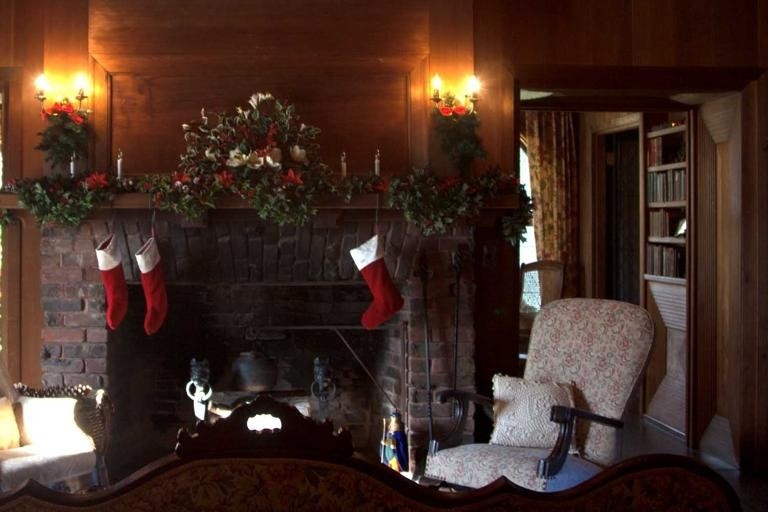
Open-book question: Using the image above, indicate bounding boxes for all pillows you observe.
[490,371,582,456]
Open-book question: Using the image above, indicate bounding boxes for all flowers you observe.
[135,89,336,226]
[42,96,91,155]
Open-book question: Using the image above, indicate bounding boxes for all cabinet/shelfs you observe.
[629,108,715,461]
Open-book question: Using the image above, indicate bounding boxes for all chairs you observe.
[429,297,659,494]
[0,370,121,498]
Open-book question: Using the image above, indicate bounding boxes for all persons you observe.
[380,413,408,473]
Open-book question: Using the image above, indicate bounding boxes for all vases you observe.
[41,148,88,180]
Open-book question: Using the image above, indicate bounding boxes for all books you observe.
[645,137,686,277]
